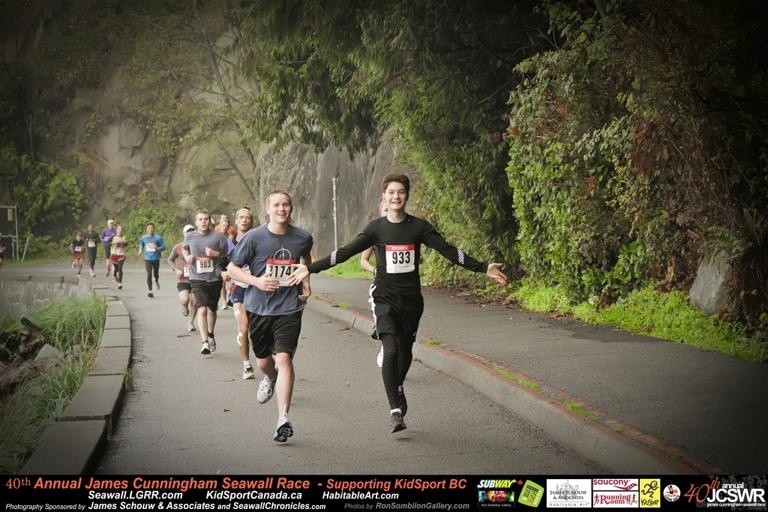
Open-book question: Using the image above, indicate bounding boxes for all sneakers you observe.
[399,387,407,416]
[201,337,216,353]
[243,367,255,380]
[257,369,277,404]
[389,411,406,434]
[273,421,293,443]
[187,323,195,331]
[222,300,228,310]
[148,292,154,297]
[182,308,188,317]
[155,282,160,291]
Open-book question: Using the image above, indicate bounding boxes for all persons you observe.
[68,209,235,355]
[285,171,508,434]
[359,199,389,368]
[215,205,255,381]
[226,188,313,444]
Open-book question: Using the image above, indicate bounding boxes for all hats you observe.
[183,223,195,232]
